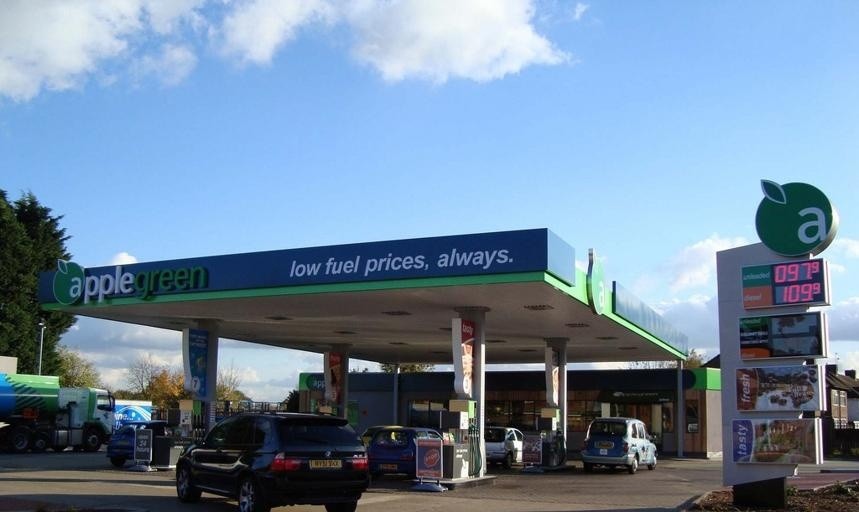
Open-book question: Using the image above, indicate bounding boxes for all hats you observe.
[486,426,523,469]
[106,420,177,467]
[176,410,370,512]
[361,425,444,477]
[580,416,657,474]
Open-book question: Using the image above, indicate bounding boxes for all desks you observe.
[38,318,48,375]
